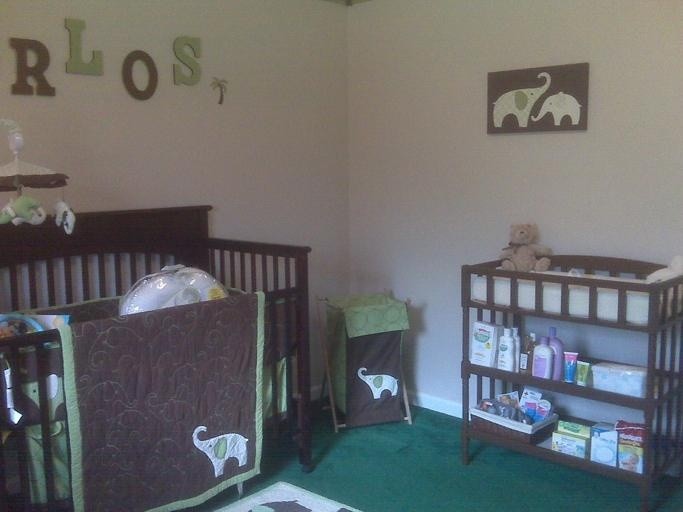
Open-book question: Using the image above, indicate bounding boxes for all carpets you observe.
[212,479,362,512]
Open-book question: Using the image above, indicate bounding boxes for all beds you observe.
[0,206,312,512]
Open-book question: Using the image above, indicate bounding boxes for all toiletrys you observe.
[562,352,579,384]
[576,360,590,386]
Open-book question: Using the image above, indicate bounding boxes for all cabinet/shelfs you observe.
[458,254,682,511]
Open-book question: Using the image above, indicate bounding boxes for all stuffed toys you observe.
[0,310,46,337]
[498,223,552,272]
[0,118,76,235]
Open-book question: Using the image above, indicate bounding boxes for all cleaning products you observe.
[497,327,564,380]
